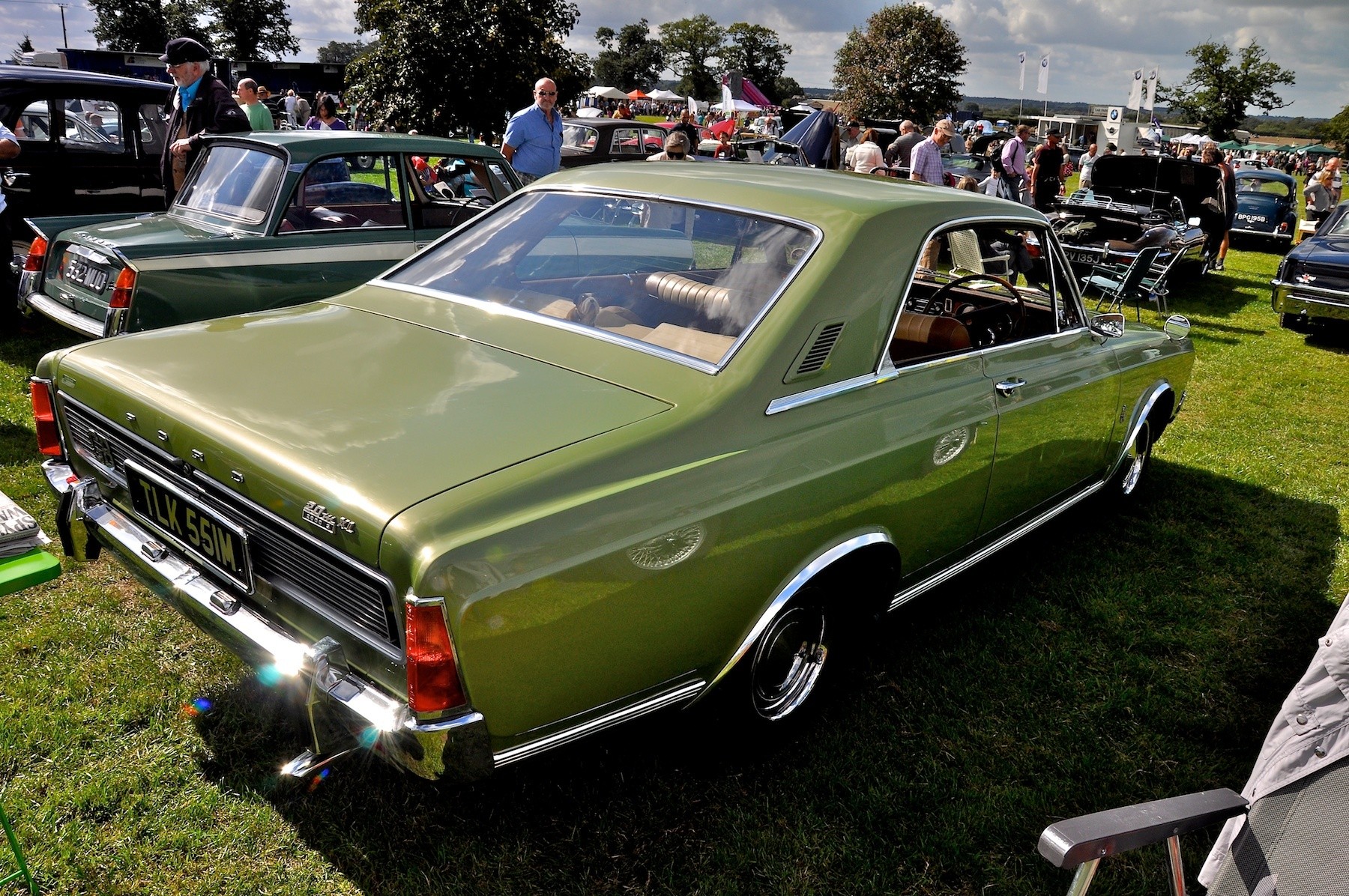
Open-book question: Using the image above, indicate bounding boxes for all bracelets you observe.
[1310,204,1313,205]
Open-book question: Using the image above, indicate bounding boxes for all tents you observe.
[710,99,763,112]
[587,86,686,104]
[1169,132,1339,156]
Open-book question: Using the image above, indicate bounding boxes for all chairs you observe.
[1037,592,1349,896]
[312,205,360,227]
[1096,245,1189,316]
[892,312,971,355]
[948,229,1014,284]
[361,219,383,227]
[643,269,751,332]
[1079,246,1162,322]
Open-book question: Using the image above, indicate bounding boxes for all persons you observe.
[304,96,347,131]
[498,77,563,187]
[1142,144,1343,272]
[280,81,301,126]
[66,99,115,143]
[311,91,327,117]
[296,96,311,126]
[15,118,26,137]
[821,107,1129,293]
[335,91,345,112]
[408,129,507,197]
[257,86,281,130]
[599,99,783,242]
[237,78,274,131]
[0,120,37,336]
[160,38,253,212]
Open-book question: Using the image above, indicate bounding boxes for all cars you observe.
[1025,148,1298,285]
[1271,200,1349,329]
[0,65,1041,341]
[31,159,1195,790]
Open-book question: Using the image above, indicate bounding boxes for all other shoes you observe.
[1215,263,1224,270]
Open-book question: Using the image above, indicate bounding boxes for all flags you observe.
[1126,67,1142,110]
[1144,68,1157,111]
[1037,54,1049,94]
[1019,51,1025,91]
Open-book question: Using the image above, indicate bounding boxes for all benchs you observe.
[212,203,294,233]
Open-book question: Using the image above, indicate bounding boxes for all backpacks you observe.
[990,140,1018,172]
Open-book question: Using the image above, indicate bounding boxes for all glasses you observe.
[539,91,557,96]
[667,150,684,159]
[847,129,854,131]
[164,63,184,68]
[1327,177,1333,180]
[939,130,951,142]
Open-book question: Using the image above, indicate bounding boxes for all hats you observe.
[1047,129,1062,137]
[158,38,210,64]
[257,86,271,97]
[90,113,103,119]
[936,119,955,138]
[847,121,860,128]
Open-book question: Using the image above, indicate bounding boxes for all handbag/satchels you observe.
[1063,156,1073,177]
